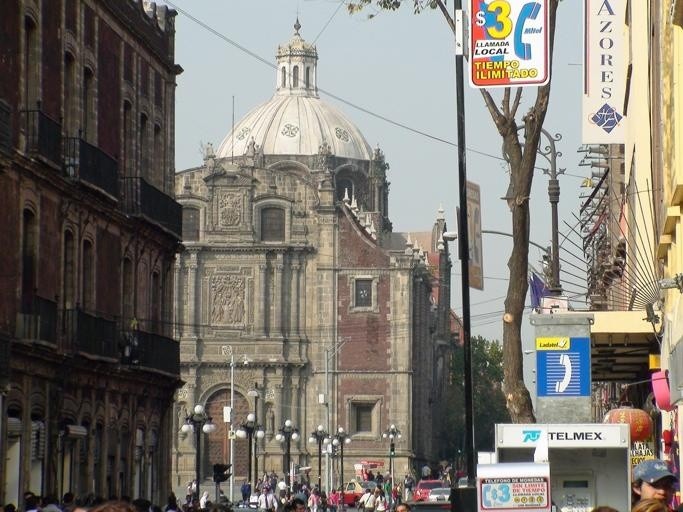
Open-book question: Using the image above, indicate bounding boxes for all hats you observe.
[633,459,680,485]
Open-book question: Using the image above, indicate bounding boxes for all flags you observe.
[529,274,533,310]
[532,272,551,309]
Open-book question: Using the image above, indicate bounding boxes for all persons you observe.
[630,499,673,511]
[630,457,678,505]
[1,460,463,512]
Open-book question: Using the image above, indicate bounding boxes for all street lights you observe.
[331,425,352,507]
[306,424,334,497]
[179,401,216,509]
[381,424,403,504]
[273,417,302,502]
[233,414,268,496]
[229,352,248,504]
[442,229,564,294]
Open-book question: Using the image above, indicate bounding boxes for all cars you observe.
[325,475,387,507]
[412,473,469,507]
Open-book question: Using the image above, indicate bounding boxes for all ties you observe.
[264,495,268,509]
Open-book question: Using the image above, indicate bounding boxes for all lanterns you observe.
[601,400,654,447]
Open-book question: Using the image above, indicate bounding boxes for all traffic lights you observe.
[390,442,396,457]
[211,462,233,482]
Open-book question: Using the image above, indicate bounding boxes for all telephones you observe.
[550,469,597,512]
